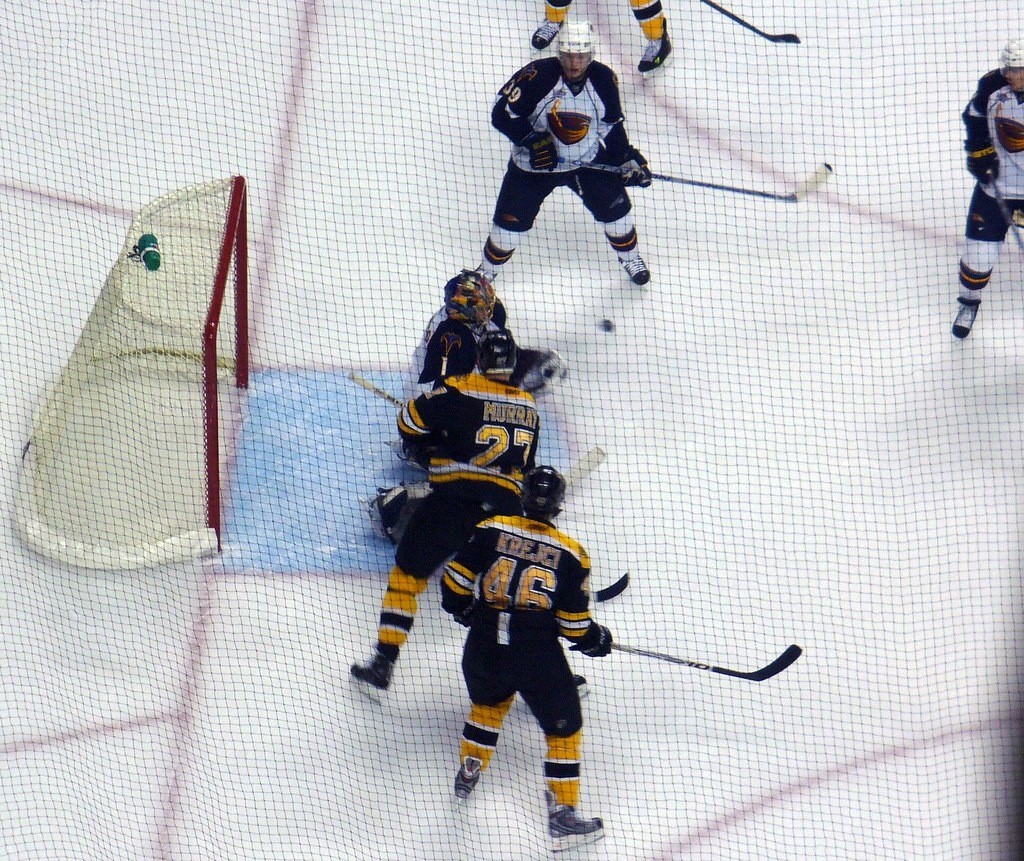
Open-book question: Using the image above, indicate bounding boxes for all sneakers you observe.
[637,34,674,77]
[351,665,391,696]
[384,437,428,471]
[546,792,605,851]
[473,261,498,283]
[454,756,482,806]
[952,304,979,342]
[573,674,591,696]
[532,19,563,53]
[360,487,407,545]
[618,254,650,290]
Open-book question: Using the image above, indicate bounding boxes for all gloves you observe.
[524,131,558,171]
[622,167,652,186]
[964,138,999,185]
[569,620,613,657]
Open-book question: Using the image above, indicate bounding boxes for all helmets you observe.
[523,465,566,517]
[444,268,495,322]
[558,21,594,53]
[476,329,516,375]
[1000,38,1024,67]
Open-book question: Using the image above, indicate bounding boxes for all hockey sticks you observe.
[986,169,1024,251]
[611,643,804,683]
[348,371,607,490]
[699,0,801,43]
[540,153,833,204]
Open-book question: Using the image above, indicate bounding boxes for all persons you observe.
[532,0,672,80]
[950,38,1024,338]
[439,466,613,852]
[409,268,566,404]
[350,330,540,704]
[464,17,653,299]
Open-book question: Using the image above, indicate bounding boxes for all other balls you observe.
[600,318,615,332]
